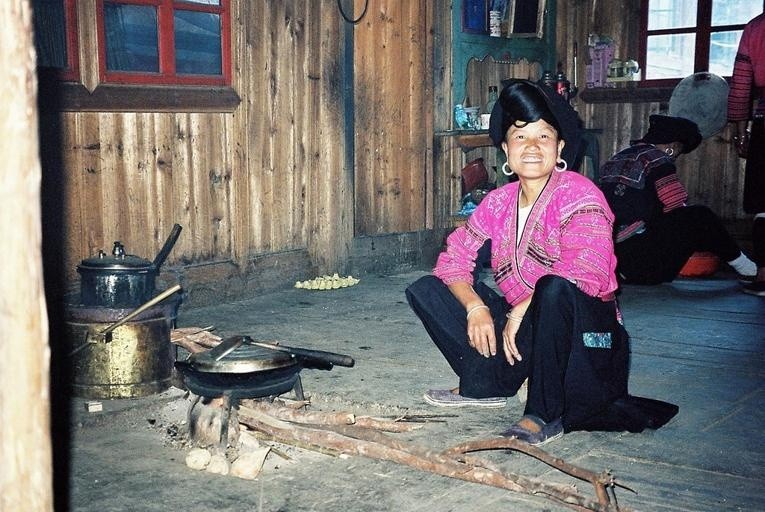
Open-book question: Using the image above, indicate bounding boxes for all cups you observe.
[480,114,492,129]
[606,57,638,76]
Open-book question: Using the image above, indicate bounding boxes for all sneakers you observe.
[739,268,765,297]
[424,387,507,409]
[498,414,564,448]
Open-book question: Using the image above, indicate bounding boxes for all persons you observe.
[405,78,680,449]
[727,11,765,297]
[598,115,759,286]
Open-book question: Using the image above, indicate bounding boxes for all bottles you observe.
[544,73,566,95]
[486,86,499,114]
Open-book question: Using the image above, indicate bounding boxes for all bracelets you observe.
[734,132,749,144]
[506,313,523,322]
[466,305,490,320]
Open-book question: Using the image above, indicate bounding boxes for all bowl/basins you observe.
[680,251,721,277]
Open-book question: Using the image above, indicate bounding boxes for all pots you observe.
[75,242,160,307]
[172,346,331,388]
